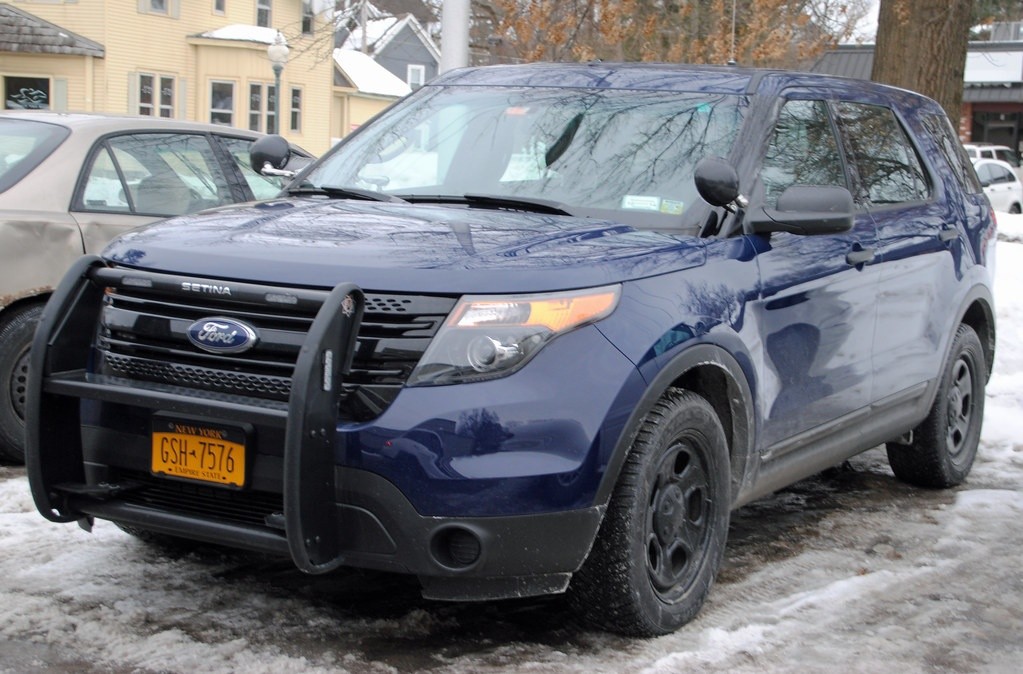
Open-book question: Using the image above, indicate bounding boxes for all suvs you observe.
[21,60,998,638]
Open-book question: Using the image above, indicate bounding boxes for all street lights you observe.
[268,29,293,136]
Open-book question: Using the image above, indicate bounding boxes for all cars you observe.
[0,110,328,467]
[962,158,1022,214]
[963,142,1021,177]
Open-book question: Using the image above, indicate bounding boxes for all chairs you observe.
[137,172,189,215]
